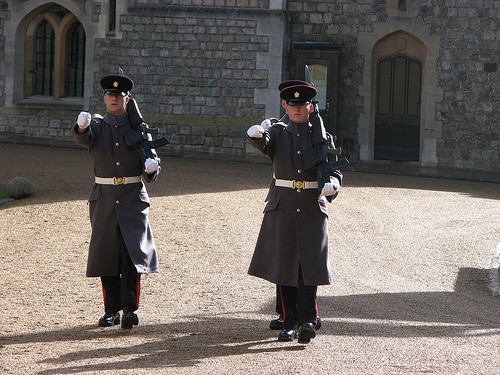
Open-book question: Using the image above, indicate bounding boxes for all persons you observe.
[246,80,343,344]
[72,74,161,328]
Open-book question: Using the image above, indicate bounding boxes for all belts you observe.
[94,176,141,185]
[273,173,277,179]
[275,179,319,189]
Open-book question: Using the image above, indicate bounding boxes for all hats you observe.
[278,80,311,91]
[100,74,134,96]
[280,86,317,105]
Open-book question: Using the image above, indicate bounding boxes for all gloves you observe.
[247,125,264,138]
[261,119,272,131]
[145,158,158,174]
[77,111,91,129]
[321,182,338,197]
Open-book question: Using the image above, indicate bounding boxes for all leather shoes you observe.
[278,329,298,341]
[270,317,284,329]
[121,313,138,329]
[99,312,120,326]
[316,318,321,330]
[298,322,315,343]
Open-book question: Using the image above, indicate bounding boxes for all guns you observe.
[119,65,170,165]
[305,64,351,188]
